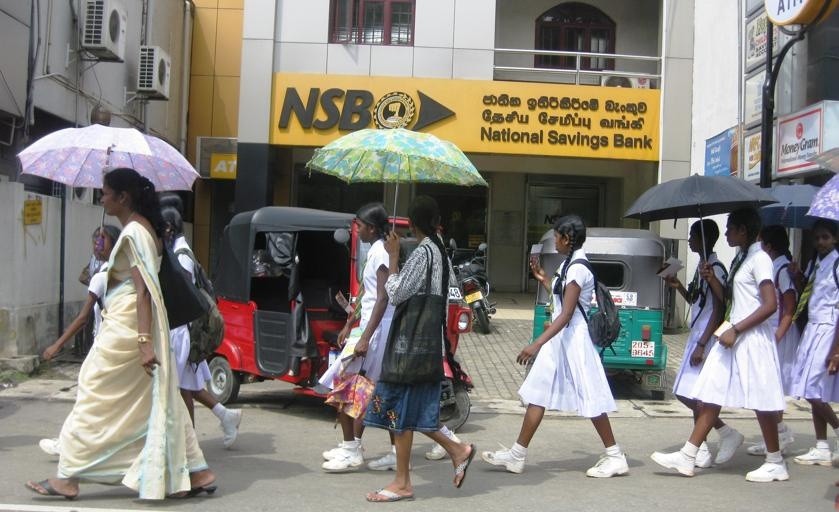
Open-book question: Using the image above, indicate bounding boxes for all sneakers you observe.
[220,410,243,447]
[369,452,411,472]
[426,434,459,460]
[746,428,793,482]
[323,443,364,470]
[483,450,524,474]
[716,428,744,464]
[39,439,62,456]
[651,451,695,477]
[794,437,839,466]
[696,448,711,467]
[586,455,628,478]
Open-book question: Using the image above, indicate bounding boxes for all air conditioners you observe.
[83,0,127,64]
[136,46,172,103]
[600,70,650,89]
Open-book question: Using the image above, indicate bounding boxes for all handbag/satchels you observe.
[563,259,621,346]
[777,263,809,329]
[175,247,224,361]
[157,241,207,329]
[379,293,447,385]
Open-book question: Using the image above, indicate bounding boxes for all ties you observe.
[355,256,369,318]
[725,255,747,321]
[792,265,818,321]
[548,268,557,313]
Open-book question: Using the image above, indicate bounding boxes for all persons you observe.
[20,169,240,500]
[650,206,839,482]
[320,197,476,500]
[481,215,629,478]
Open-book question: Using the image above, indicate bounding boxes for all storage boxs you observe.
[455,233,485,248]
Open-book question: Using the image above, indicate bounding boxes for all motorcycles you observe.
[453,241,497,336]
[528,225,668,403]
[210,208,475,431]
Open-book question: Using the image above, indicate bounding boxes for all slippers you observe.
[24,481,78,501]
[366,488,414,502]
[172,488,217,500]
[453,444,477,488]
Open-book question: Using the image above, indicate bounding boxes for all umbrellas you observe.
[805,173,839,222]
[304,125,490,234]
[755,182,823,267]
[15,123,203,255]
[622,174,781,263]
